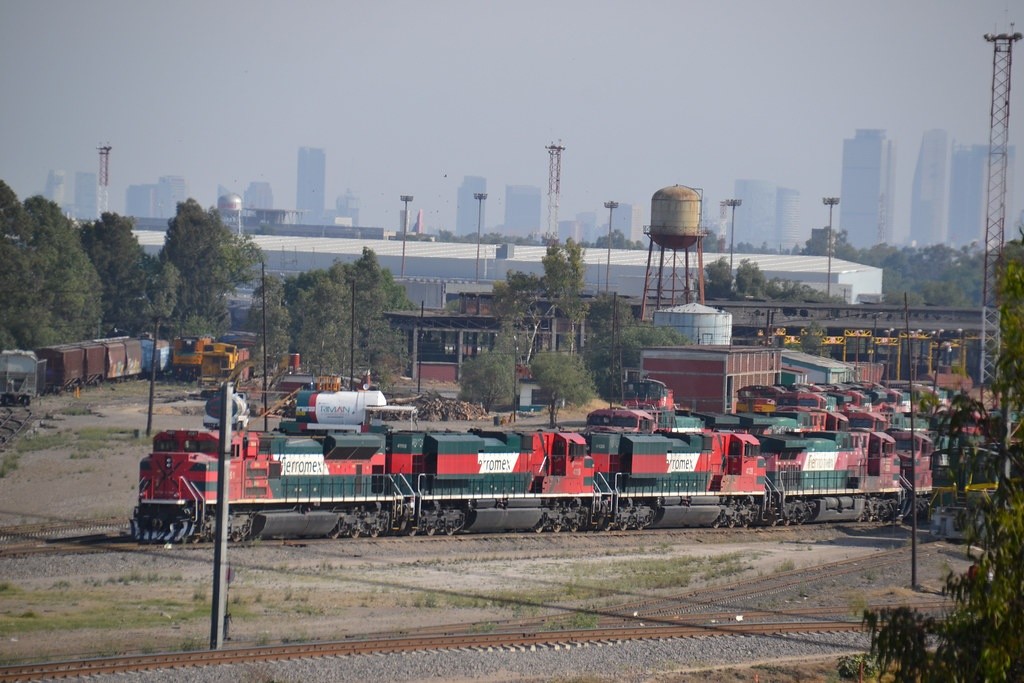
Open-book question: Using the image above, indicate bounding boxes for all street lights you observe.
[474,192,488,283]
[604,200,619,295]
[724,199,743,294]
[824,196,841,299]
[399,194,414,278]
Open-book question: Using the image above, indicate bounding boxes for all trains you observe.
[127,378,1024,545]
[0,331,172,409]
